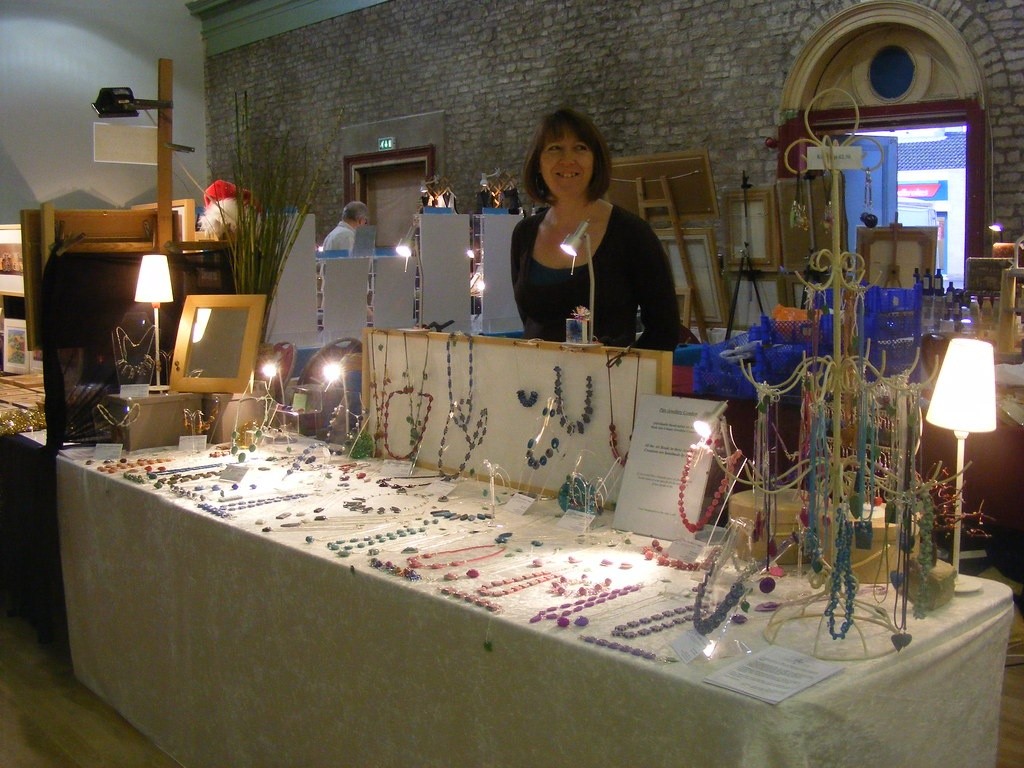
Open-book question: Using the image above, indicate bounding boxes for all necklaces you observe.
[690,286,920,651]
[87,325,741,661]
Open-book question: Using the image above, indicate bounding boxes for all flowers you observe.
[572,305,592,323]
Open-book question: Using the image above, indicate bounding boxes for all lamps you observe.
[324,351,353,434]
[261,351,286,428]
[479,172,488,187]
[91,86,174,119]
[988,223,1005,242]
[419,178,429,194]
[396,225,418,257]
[133,254,175,391]
[558,219,596,343]
[924,336,997,593]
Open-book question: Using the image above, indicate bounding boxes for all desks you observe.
[670,366,1024,583]
[56,444,1013,768]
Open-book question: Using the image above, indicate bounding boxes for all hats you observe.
[197,179,266,240]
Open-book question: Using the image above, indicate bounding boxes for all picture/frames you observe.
[131,199,195,253]
[3,318,30,375]
[786,273,851,309]
[857,225,937,288]
[655,227,730,329]
[168,294,268,392]
[0,224,24,297]
[722,272,786,331]
[606,147,720,222]
[724,184,780,272]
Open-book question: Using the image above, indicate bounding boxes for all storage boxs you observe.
[692,282,921,400]
[819,510,920,583]
[205,393,269,445]
[108,391,204,451]
[0,372,45,415]
[728,488,809,563]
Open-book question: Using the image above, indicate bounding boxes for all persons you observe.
[322,201,369,251]
[203,179,261,242]
[511,109,679,351]
